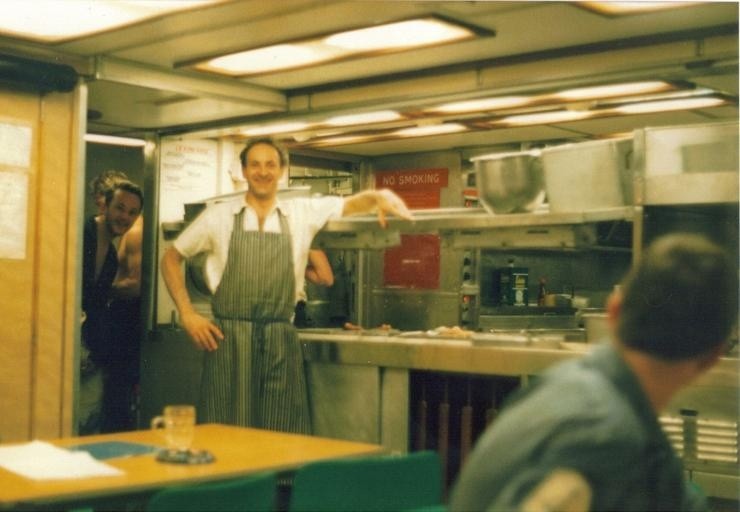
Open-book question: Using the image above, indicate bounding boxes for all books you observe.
[541,136,633,212]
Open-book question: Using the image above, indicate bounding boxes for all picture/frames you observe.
[0,423,386,512]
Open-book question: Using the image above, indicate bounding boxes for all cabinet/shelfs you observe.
[538,276,547,307]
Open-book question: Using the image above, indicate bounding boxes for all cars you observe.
[151,405,197,453]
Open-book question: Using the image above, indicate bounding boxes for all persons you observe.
[293,248,337,327]
[445,232,737,511]
[157,136,416,431]
[77,179,146,437]
[88,169,147,436]
